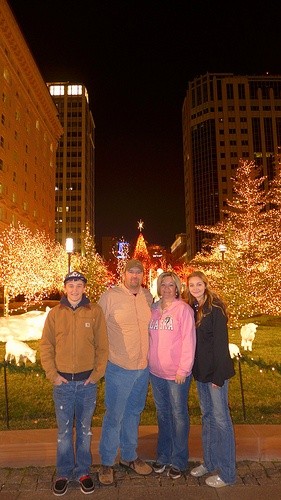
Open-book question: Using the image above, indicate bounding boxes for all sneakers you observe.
[119,457,153,475]
[98,465,114,485]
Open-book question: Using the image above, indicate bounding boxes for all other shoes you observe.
[79,475,95,494]
[169,468,182,479]
[191,465,208,477]
[153,463,166,473]
[53,477,69,496]
[205,475,229,488]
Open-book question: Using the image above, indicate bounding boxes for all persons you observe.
[147,271,196,479]
[181,272,237,488]
[97,259,154,485]
[39,271,109,496]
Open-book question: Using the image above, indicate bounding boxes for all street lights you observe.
[219,243,227,262]
[66,237,74,275]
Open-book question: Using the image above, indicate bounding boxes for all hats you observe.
[126,259,143,271]
[63,271,85,282]
